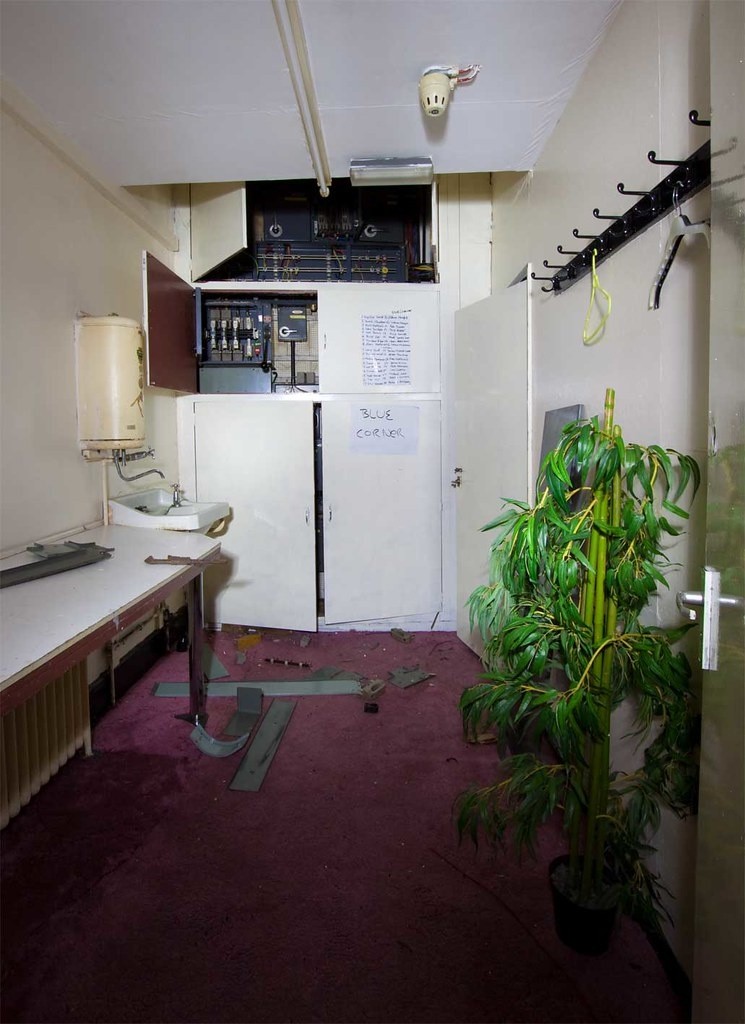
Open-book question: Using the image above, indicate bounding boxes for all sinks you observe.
[108,487,230,536]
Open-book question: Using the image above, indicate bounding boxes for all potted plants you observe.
[450,387,703,950]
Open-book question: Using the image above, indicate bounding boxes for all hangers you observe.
[581,249,612,344]
[648,180,712,307]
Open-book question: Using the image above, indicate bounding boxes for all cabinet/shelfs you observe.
[140,179,539,673]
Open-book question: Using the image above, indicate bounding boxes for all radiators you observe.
[0,657,95,831]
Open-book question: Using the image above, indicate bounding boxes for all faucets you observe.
[111,444,166,482]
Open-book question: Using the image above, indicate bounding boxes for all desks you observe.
[0,524,223,731]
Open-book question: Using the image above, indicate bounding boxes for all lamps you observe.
[349,156,433,187]
